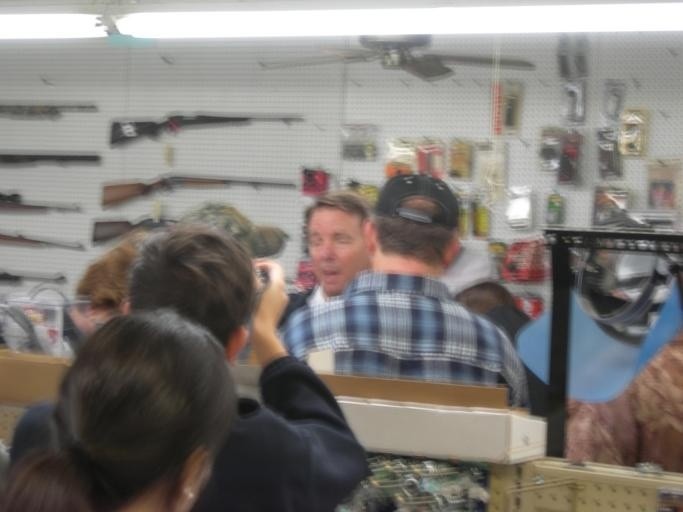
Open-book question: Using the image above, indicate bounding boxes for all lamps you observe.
[111,2,681,41]
[3,12,111,39]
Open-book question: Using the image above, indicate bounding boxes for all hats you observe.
[512,258,683,404]
[373,172,459,228]
[179,205,285,257]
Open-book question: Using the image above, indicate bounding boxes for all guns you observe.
[0,104,98,119]
[100,175,295,209]
[90,216,288,247]
[0,232,82,252]
[0,272,66,285]
[0,150,100,165]
[110,113,306,143]
[0,194,80,218]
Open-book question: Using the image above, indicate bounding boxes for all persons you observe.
[563,89,583,120]
[133,222,375,512]
[450,278,566,417]
[2,311,243,511]
[1,233,156,369]
[167,203,290,312]
[275,192,379,333]
[606,91,622,120]
[274,171,534,413]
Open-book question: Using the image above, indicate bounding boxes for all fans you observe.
[256,35,535,85]
